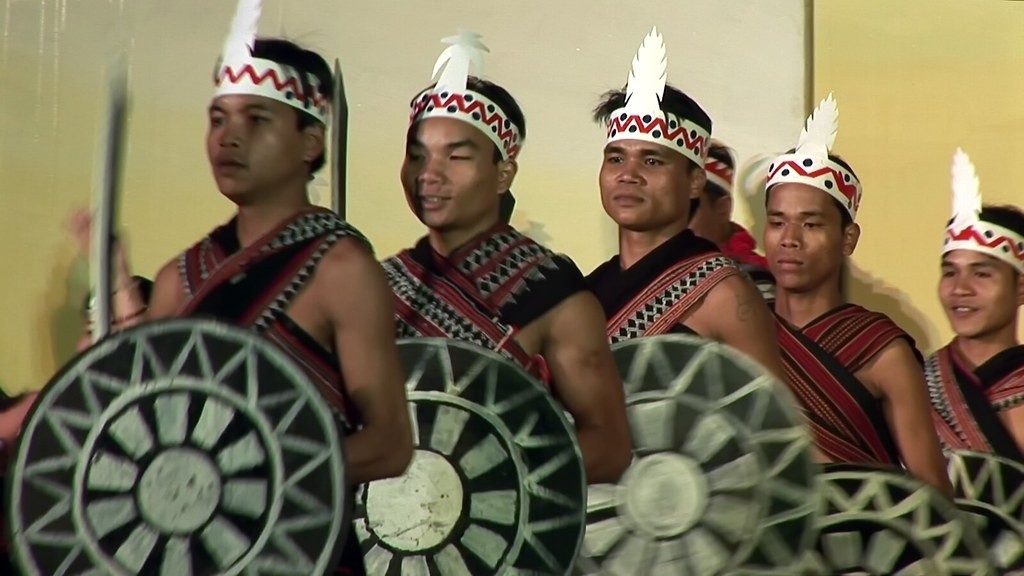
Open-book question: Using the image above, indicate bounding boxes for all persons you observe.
[764,93,954,500]
[688,138,778,313]
[584,25,784,386]
[379,27,632,482]
[0,0,413,576]
[922,145,1024,467]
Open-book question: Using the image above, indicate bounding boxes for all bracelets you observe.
[111,306,149,325]
[110,280,141,296]
